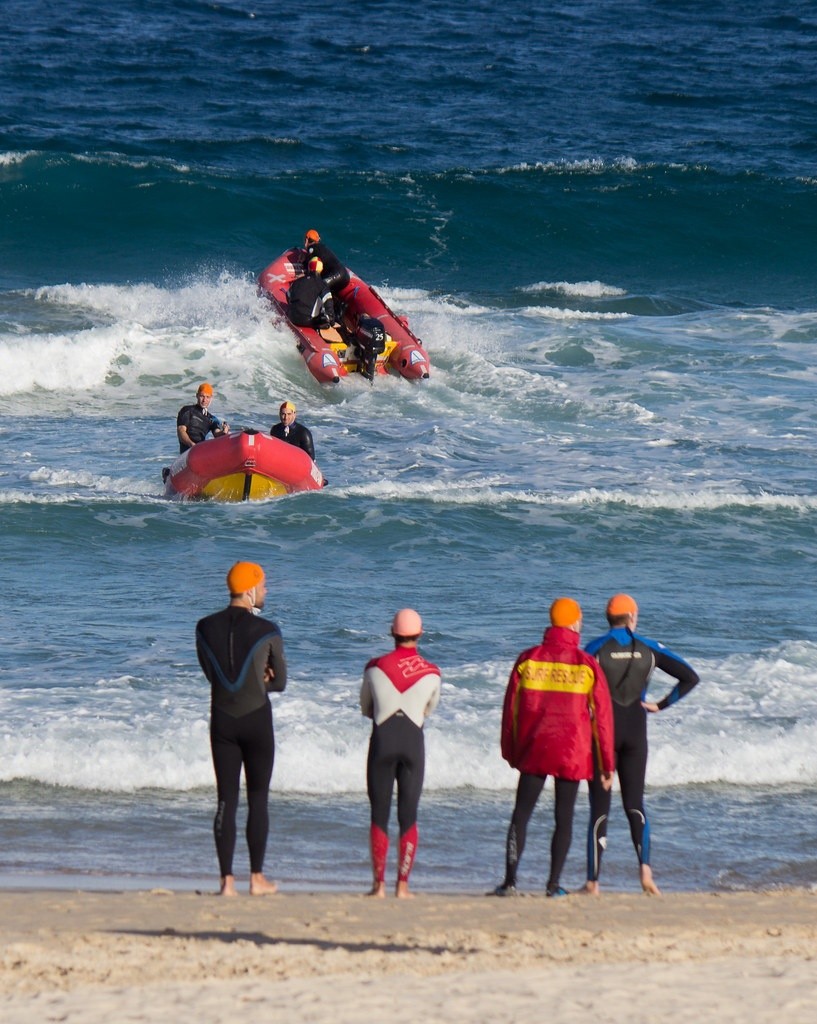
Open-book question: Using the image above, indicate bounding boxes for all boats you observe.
[161,427,330,504]
[257,246,431,385]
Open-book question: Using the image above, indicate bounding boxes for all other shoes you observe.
[546,886,570,896]
[494,885,516,896]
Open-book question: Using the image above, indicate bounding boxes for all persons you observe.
[360,610,441,899]
[574,594,700,898]
[270,402,315,462]
[196,561,287,896]
[287,230,350,329]
[177,384,230,455]
[485,597,614,897]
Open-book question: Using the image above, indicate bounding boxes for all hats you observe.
[307,256,324,274]
[549,597,582,628]
[391,608,422,638]
[361,313,370,318]
[198,383,213,396]
[305,230,320,242]
[280,401,296,412]
[226,561,264,594]
[606,594,638,615]
